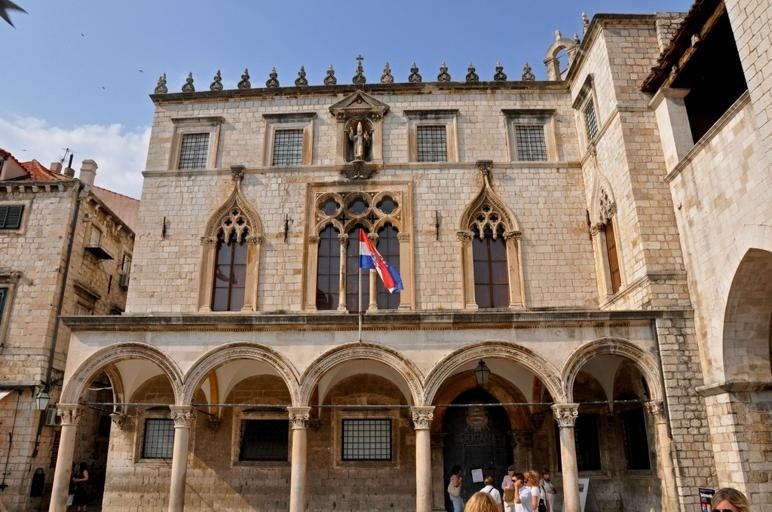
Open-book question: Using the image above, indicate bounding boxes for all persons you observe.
[71,462,89,512]
[711,489,752,512]
[448,465,558,512]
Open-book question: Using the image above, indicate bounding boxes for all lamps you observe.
[33,379,64,409]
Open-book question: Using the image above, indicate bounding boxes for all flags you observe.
[359,227,404,294]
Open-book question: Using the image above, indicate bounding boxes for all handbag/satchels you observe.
[538,499,546,512]
[447,482,461,496]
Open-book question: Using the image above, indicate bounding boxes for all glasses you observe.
[512,480,515,483]
[524,479,528,483]
[711,509,733,512]
